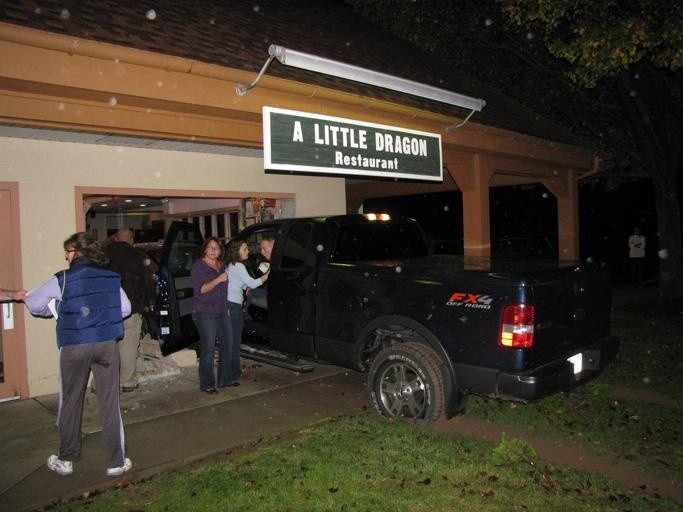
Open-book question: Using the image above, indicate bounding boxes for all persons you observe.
[260,238,274,261]
[90,228,152,395]
[25,231,134,477]
[225,239,268,382]
[627,225,649,291]
[190,237,239,393]
[0,287,28,303]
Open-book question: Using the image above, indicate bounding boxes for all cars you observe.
[130,240,207,339]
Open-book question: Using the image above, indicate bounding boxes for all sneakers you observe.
[200,388,218,395]
[44,453,75,477]
[106,456,133,478]
[121,383,139,393]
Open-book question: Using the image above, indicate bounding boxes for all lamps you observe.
[233,44,488,134]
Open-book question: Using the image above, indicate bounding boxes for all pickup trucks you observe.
[146,211,619,422]
